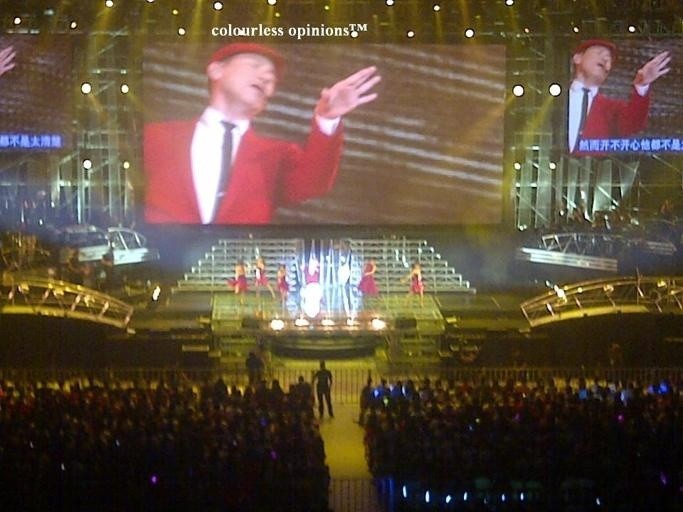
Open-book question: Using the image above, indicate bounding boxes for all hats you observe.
[573,39,619,52]
[207,42,284,83]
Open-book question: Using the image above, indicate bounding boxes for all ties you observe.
[573,88,590,153]
[211,121,236,221]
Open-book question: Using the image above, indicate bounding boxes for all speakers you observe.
[242,318,259,328]
[395,318,416,328]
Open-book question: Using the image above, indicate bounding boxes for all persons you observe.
[399,262,425,308]
[146,42,383,224]
[361,367,683,512]
[522,193,683,273]
[299,255,326,306]
[311,359,337,420]
[277,262,288,303]
[0,229,116,297]
[225,255,249,300]
[253,254,277,301]
[0,45,17,77]
[245,352,264,388]
[0,367,328,512]
[565,39,672,157]
[355,255,384,304]
